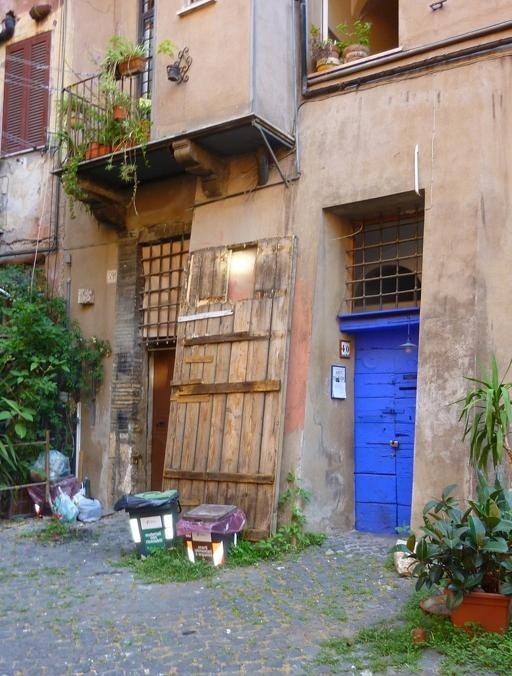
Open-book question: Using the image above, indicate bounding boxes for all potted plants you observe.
[387,355,512,635]
[47,34,182,220]
[310,15,373,74]
